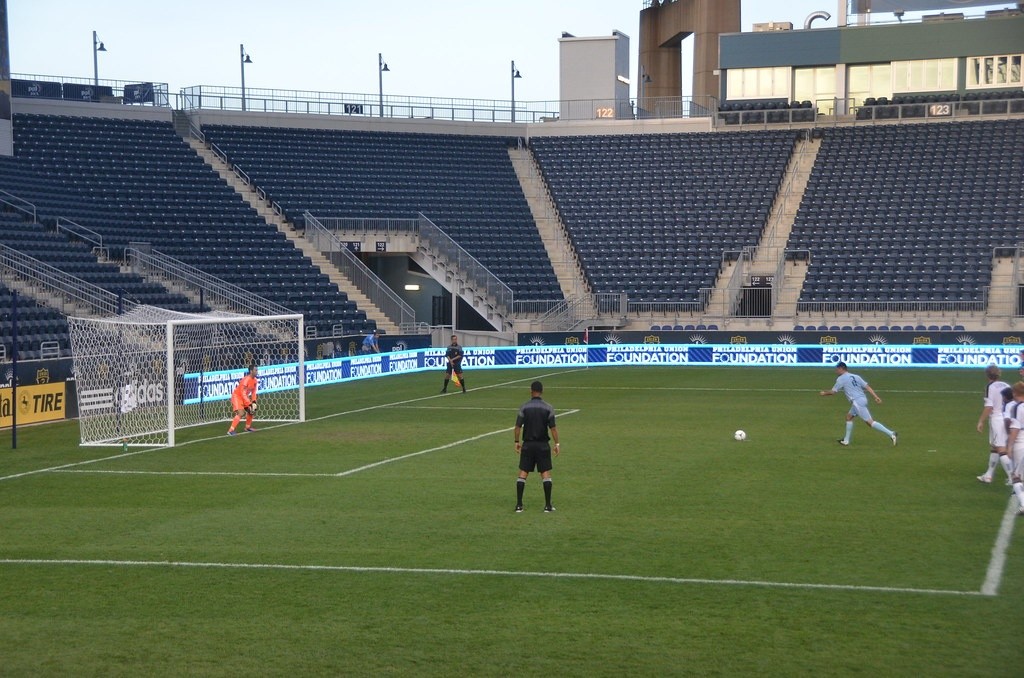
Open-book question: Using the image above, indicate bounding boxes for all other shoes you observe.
[463,389,466,393]
[244,427,257,433]
[440,389,446,393]
[226,431,237,437]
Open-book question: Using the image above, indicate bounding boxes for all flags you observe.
[451,368,461,388]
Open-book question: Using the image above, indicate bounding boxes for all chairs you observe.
[0,92,1024,363]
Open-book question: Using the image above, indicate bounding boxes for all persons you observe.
[820,362,898,446]
[514,380,560,513]
[977,365,1024,514]
[362,330,381,355]
[441,335,467,394]
[227,364,259,436]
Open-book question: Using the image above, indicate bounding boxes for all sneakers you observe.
[544,507,556,512]
[1014,507,1024,515]
[977,475,991,483]
[891,432,898,446]
[515,507,523,512]
[837,439,850,446]
[1004,478,1013,485]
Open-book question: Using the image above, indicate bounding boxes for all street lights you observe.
[240,53,254,113]
[379,63,392,116]
[512,71,522,124]
[640,74,654,111]
[94,40,107,101]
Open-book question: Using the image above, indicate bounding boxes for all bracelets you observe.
[515,441,519,443]
[555,443,559,446]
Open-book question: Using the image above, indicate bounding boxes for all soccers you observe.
[734,430,746,441]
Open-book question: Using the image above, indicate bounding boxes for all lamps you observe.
[405,284,420,291]
